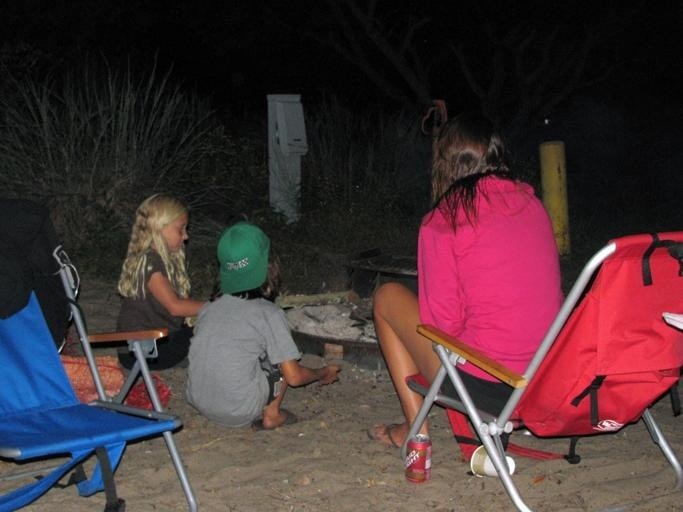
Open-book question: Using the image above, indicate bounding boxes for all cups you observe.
[468,445,515,476]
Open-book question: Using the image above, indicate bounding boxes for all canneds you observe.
[405,434,432,483]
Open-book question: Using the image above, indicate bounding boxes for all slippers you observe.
[252,408,298,432]
[367,424,399,448]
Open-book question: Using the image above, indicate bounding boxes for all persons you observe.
[366,110,566,460]
[185,222,340,432]
[112,192,209,372]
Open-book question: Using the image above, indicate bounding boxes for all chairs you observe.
[399,228,682,512]
[0,195,207,510]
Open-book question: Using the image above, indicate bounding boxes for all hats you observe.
[217,223,271,295]
